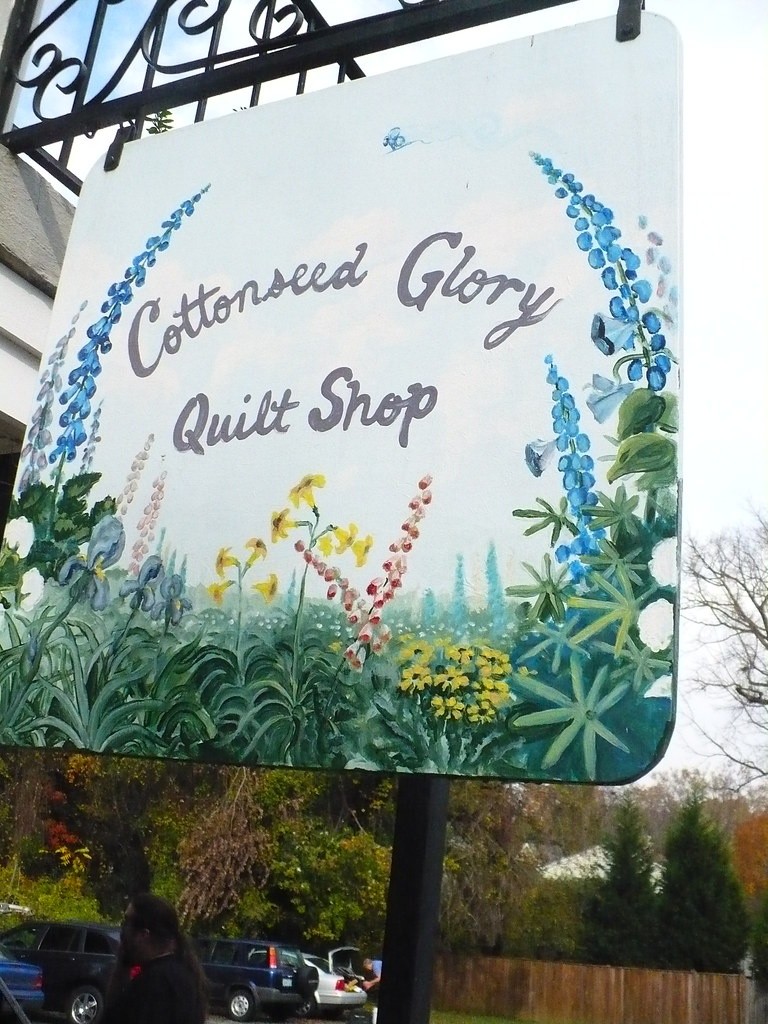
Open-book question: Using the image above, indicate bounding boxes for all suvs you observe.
[0,920,143,1024]
[189,937,320,1024]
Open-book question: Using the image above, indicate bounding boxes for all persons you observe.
[104,893,210,1024]
[363,958,383,991]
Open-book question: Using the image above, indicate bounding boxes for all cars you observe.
[246,945,367,1017]
